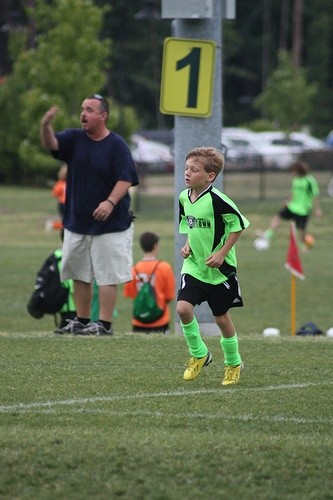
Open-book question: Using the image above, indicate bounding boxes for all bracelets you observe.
[107,199,114,207]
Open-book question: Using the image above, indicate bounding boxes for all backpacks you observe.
[131,261,166,323]
[28,253,70,319]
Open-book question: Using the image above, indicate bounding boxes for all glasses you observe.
[93,94,106,105]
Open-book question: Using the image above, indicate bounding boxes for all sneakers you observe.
[182,352,212,380]
[53,317,89,334]
[74,321,113,335]
[221,363,244,386]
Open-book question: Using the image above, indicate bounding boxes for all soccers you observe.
[251,236,270,252]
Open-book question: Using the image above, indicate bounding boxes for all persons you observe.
[53,229,77,329]
[42,96,139,336]
[255,159,319,250]
[90,280,118,323]
[124,232,176,334]
[176,148,250,384]
[52,166,66,221]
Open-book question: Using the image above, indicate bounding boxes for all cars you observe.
[222,127,333,168]
[130,129,175,162]
